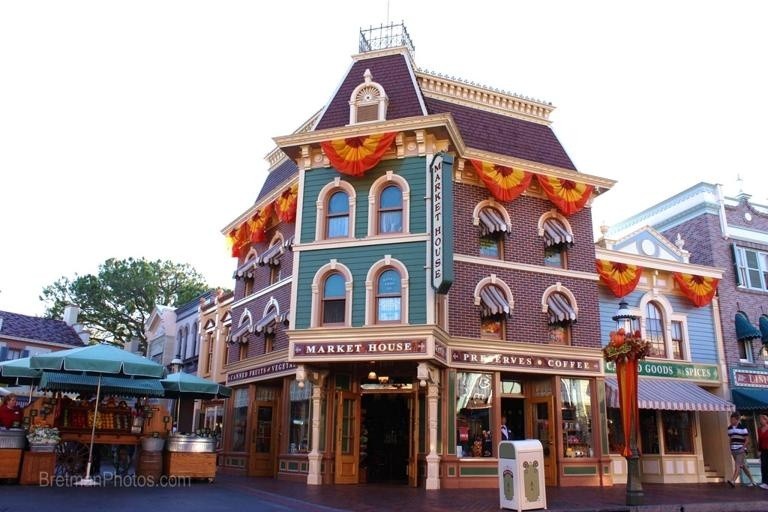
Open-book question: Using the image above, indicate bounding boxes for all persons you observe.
[194,424,221,449]
[728,411,757,487]
[500,415,513,440]
[0,394,23,428]
[472,429,492,457]
[172,421,177,432]
[756,414,768,492]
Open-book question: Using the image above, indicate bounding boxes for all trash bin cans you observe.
[497,439,547,512]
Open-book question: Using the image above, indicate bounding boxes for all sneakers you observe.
[758,483,767,489]
[746,483,756,487]
[727,480,735,487]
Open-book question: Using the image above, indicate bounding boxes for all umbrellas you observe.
[160,367,233,433]
[0,353,42,403]
[35,340,169,478]
